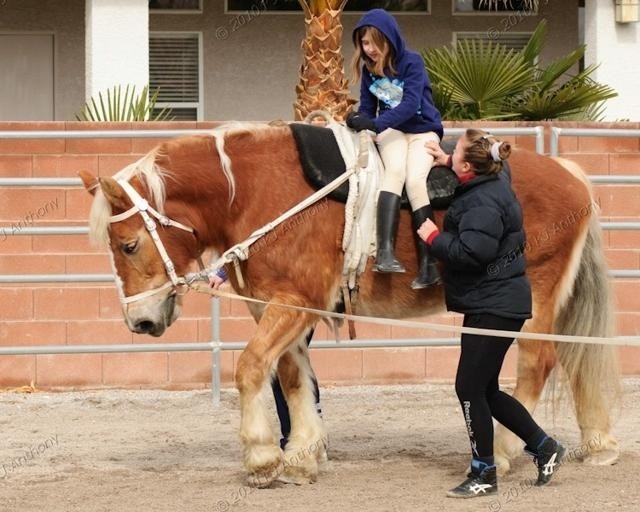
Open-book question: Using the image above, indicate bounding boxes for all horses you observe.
[75,119,627,489]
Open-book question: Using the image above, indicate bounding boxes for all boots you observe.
[410,204,445,290]
[444,455,501,499]
[371,190,407,274]
[522,427,567,487]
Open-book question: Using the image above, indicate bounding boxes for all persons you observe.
[412,126,568,500]
[344,8,447,287]
